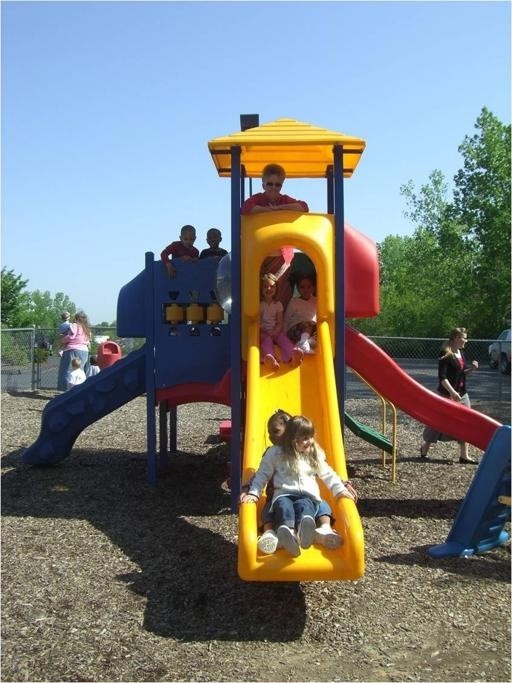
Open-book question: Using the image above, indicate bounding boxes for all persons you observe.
[60,308,103,394]
[160,222,199,317]
[285,275,321,352]
[247,415,357,556]
[241,164,310,308]
[243,407,360,542]
[260,272,302,371]
[198,228,226,325]
[418,325,479,465]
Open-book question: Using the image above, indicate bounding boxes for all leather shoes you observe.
[458,456,479,465]
[420,445,430,460]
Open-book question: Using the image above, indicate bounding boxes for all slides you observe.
[345,323,505,450]
[239,209,367,581]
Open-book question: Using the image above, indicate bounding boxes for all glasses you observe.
[264,181,283,187]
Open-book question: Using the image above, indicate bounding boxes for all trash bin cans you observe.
[97,341,121,369]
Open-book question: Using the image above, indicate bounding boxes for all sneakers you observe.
[265,353,280,371]
[299,515,316,550]
[289,346,304,369]
[295,335,317,355]
[314,526,345,549]
[257,532,278,555]
[276,524,301,558]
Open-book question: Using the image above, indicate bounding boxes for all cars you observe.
[26,334,55,356]
[488,328,511,373]
[92,334,139,351]
